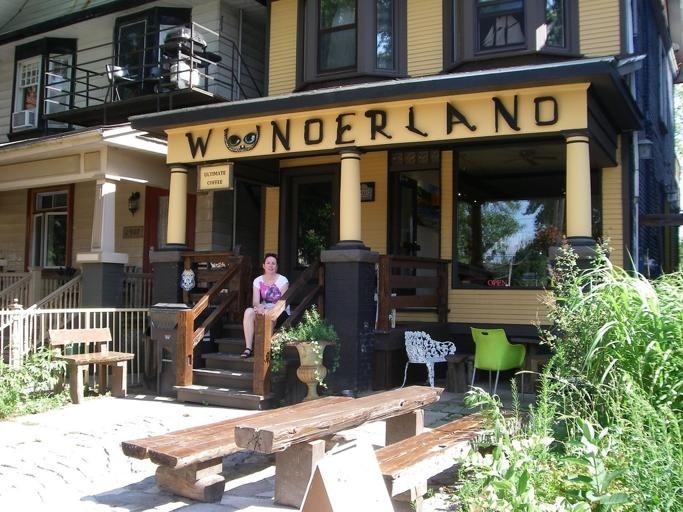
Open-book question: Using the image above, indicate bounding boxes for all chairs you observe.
[101,63,140,103]
[397,323,528,403]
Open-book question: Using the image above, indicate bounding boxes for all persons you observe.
[237,253,290,359]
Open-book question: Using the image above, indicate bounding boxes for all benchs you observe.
[47,325,138,405]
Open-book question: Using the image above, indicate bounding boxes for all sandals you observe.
[240,348,253,358]
